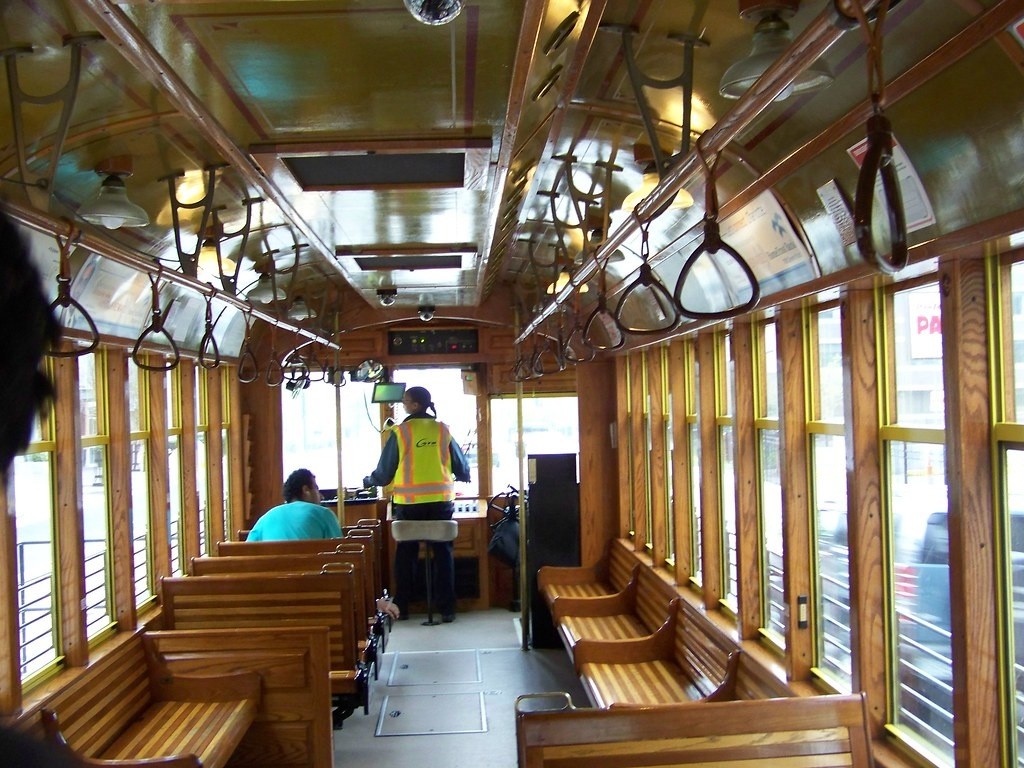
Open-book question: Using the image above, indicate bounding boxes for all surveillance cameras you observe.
[376,287,399,308]
[403,0,465,26]
[418,292,437,321]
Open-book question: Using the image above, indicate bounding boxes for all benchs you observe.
[3,518,396,768]
[515,541,874,768]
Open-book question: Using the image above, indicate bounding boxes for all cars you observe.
[461,443,500,467]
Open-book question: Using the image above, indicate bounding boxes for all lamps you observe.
[546,263,589,294]
[622,162,694,211]
[286,295,317,320]
[576,230,626,264]
[718,8,836,99]
[74,173,150,230]
[196,239,236,274]
[245,273,287,303]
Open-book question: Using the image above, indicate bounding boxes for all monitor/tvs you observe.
[372,382,406,403]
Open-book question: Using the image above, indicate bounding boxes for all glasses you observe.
[402,399,412,403]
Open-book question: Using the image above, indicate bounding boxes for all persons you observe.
[362,385,472,624]
[243,468,400,620]
